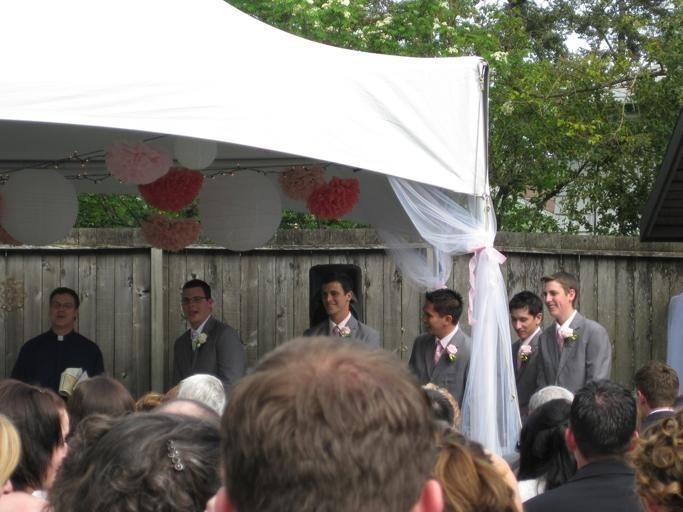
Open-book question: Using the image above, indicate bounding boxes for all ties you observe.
[332,326,341,336]
[191,331,198,351]
[556,328,565,353]
[516,348,523,369]
[433,341,442,366]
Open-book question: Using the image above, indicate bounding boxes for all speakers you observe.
[309,264,362,327]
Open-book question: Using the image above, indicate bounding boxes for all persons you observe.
[511,273,611,428]
[408,288,472,440]
[303,273,380,352]
[174,279,249,404]
[1,335,682,511]
[11,287,104,405]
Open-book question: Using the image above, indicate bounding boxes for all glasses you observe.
[182,296,206,306]
